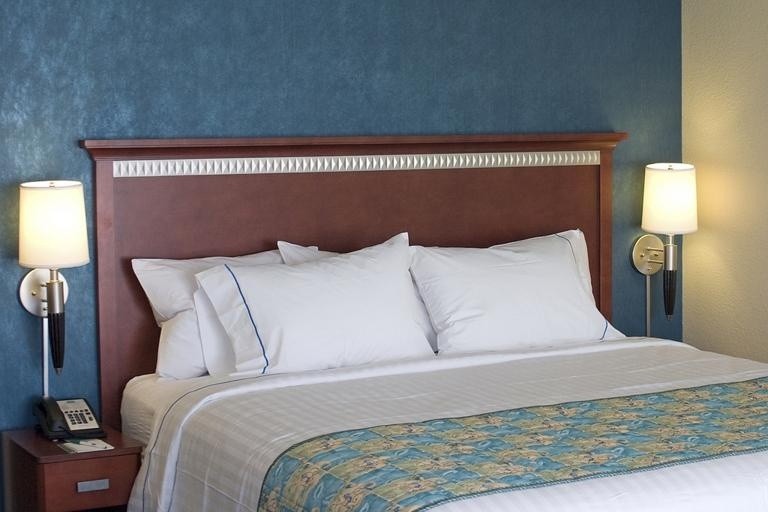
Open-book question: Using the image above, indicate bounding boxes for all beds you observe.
[79,131,766,512]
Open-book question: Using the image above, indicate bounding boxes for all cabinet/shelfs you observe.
[0,426,148,512]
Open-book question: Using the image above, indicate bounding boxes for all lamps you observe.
[630,161,699,338]
[14,177,91,401]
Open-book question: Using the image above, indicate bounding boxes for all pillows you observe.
[408,228,624,361]
[131,244,318,376]
[201,244,436,377]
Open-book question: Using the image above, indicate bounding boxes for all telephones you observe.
[33,396,107,439]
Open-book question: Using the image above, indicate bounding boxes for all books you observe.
[55,438,115,454]
